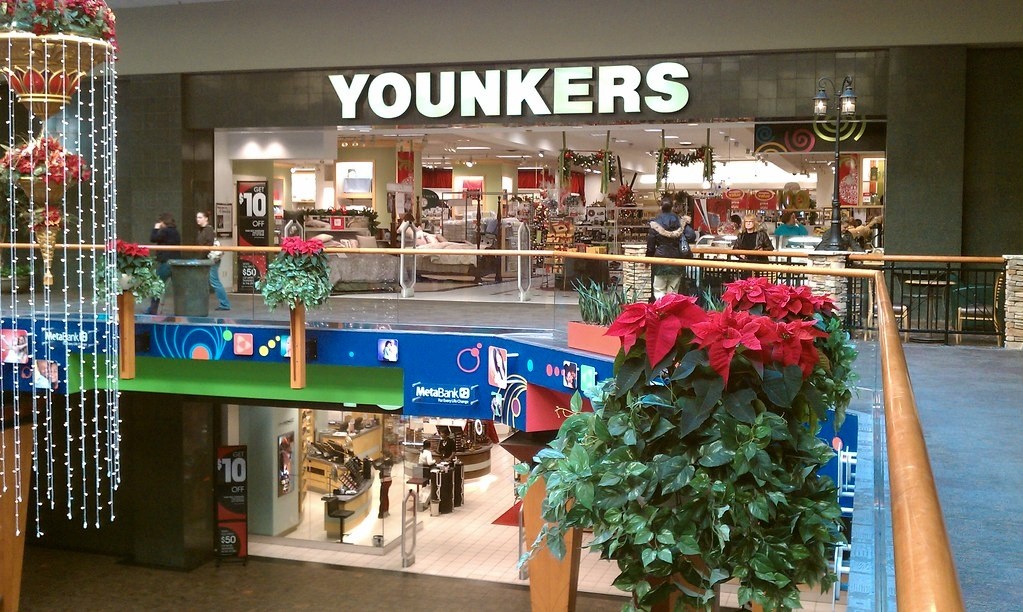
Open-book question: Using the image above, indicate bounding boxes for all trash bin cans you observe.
[168,259,211,317]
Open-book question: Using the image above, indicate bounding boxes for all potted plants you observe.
[0,183,39,295]
[564,275,647,358]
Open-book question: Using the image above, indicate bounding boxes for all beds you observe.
[440,214,518,243]
[302,214,402,293]
[389,194,504,286]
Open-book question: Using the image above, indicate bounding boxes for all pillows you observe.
[416,230,423,239]
[416,239,425,246]
[313,233,333,243]
[427,235,438,243]
[355,234,378,248]
[339,238,350,248]
[323,239,346,247]
[436,233,448,242]
[349,239,359,248]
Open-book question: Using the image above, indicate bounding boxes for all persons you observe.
[733,214,775,279]
[644,203,696,301]
[195,212,231,311]
[340,416,352,432]
[143,213,180,314]
[822,214,862,252]
[369,449,394,518]
[354,417,364,430]
[419,441,439,502]
[773,212,809,248]
[439,428,455,462]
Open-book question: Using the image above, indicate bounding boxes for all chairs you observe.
[954,271,1004,343]
[866,270,911,344]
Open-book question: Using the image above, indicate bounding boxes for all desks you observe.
[902,279,961,343]
[407,477,429,512]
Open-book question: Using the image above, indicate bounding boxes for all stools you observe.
[326,496,356,545]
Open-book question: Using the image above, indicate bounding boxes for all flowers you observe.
[255,234,331,310]
[19,202,78,231]
[91,240,168,302]
[0,125,96,187]
[0,0,122,61]
[488,268,857,612]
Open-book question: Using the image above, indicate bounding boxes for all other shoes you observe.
[214,306,231,311]
[432,499,440,503]
[144,310,157,315]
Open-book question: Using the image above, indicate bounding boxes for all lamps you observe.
[360,136,367,146]
[538,151,544,158]
[370,135,377,146]
[341,138,349,147]
[443,143,478,168]
[351,137,360,146]
[648,136,811,179]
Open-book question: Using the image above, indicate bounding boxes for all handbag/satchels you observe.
[207,236,224,261]
[676,215,693,259]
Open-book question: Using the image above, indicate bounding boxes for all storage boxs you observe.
[639,233,647,242]
[586,247,608,254]
[632,232,640,242]
[343,177,371,193]
[575,243,587,253]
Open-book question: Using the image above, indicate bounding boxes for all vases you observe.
[0,29,118,74]
[114,269,144,292]
[16,173,80,205]
[33,226,61,284]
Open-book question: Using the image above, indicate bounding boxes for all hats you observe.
[438,427,450,436]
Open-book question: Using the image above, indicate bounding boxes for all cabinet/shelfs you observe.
[570,215,616,252]
[616,206,685,257]
[539,242,567,286]
